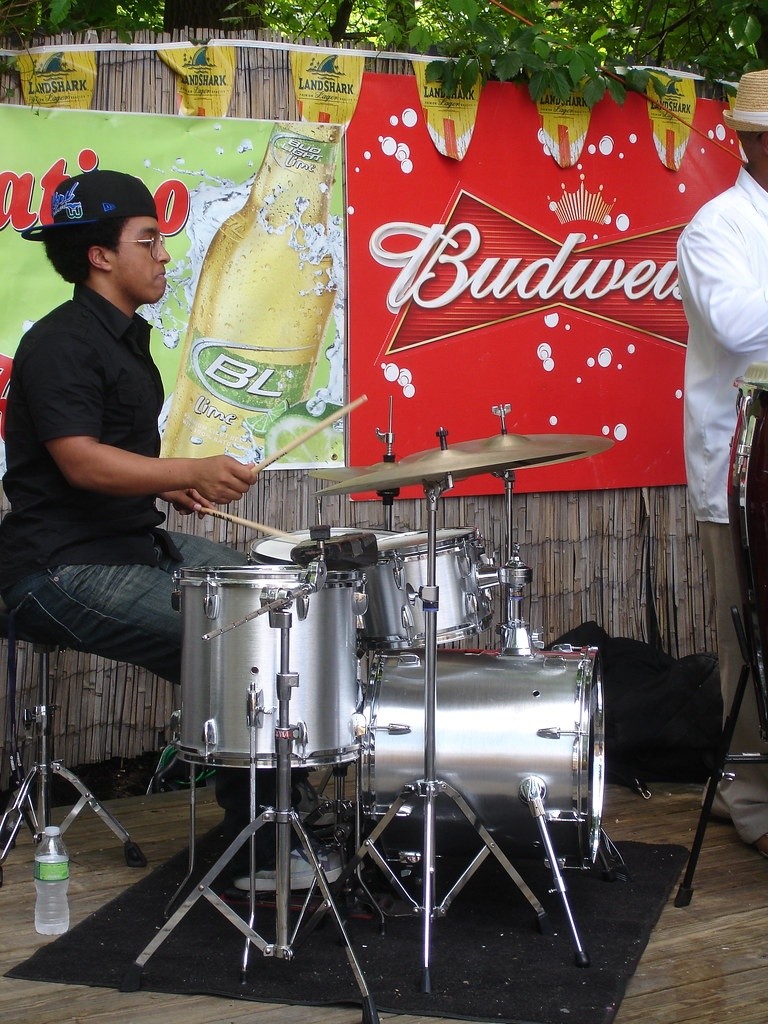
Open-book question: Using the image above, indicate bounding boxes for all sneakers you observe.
[297,782,334,825]
[235,846,347,891]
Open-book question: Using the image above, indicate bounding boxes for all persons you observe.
[674,67,767,858]
[0,169,345,894]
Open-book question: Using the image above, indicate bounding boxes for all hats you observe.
[21,171,158,241]
[722,68,768,131]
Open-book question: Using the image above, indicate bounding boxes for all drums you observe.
[724,359,767,651]
[344,646,628,898]
[347,522,505,655]
[160,556,386,777]
[241,518,413,575]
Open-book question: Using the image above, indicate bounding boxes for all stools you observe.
[0,597,149,887]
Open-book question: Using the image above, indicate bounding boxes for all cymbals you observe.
[301,422,615,500]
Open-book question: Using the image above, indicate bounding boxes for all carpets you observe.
[3,815,693,1024]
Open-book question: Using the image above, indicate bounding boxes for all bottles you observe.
[34,826,70,935]
[151,122,342,464]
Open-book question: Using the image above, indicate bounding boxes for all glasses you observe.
[119,234,165,260]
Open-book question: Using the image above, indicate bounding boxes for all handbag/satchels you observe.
[541,620,724,800]
